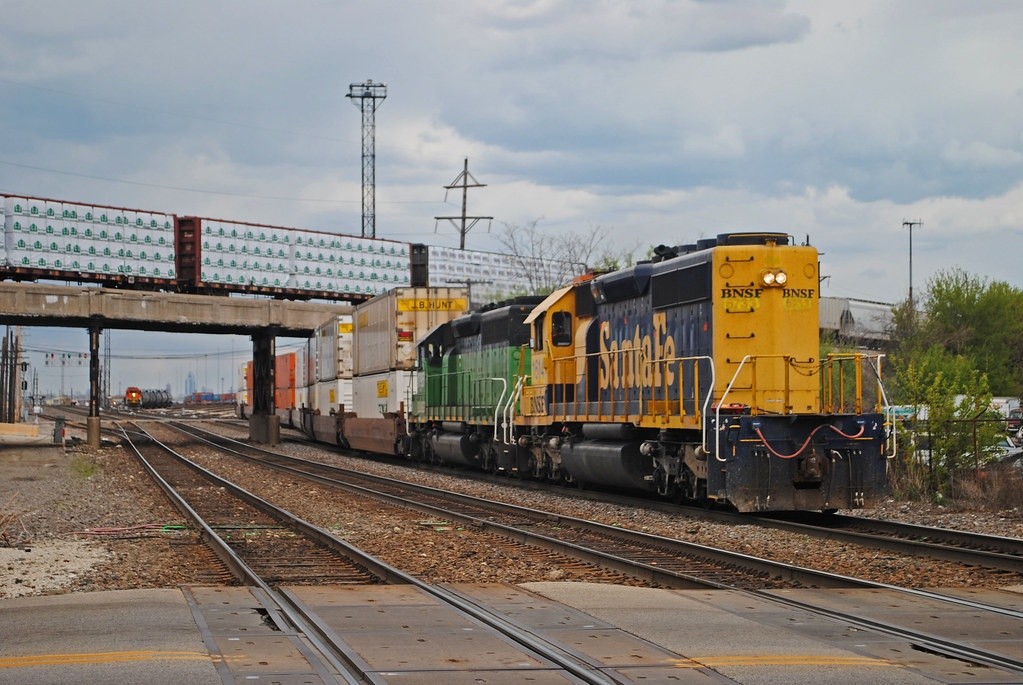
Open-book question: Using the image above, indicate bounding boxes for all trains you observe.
[126,387,172,408]
[234,230,896,516]
[184,393,238,405]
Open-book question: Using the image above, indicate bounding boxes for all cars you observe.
[1004,409,1023,432]
[910,433,1023,510]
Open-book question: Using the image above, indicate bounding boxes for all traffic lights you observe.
[45,352,87,358]
